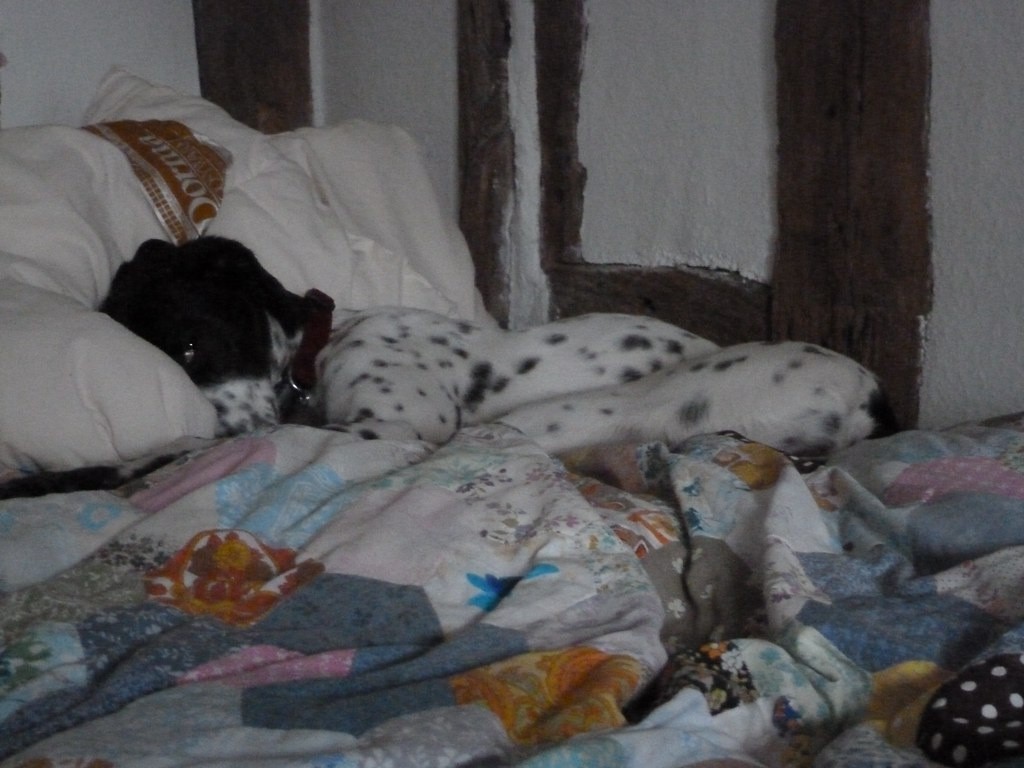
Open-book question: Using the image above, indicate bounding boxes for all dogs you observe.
[92,236,902,474]
